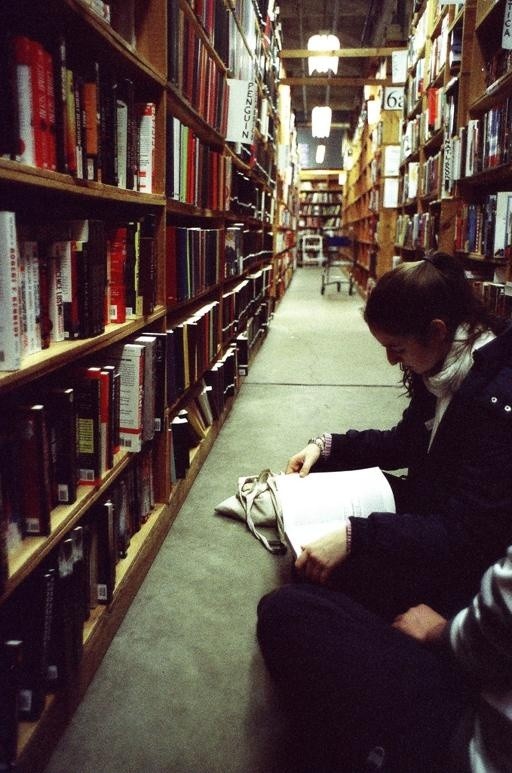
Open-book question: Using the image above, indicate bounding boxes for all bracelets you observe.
[308,439,324,459]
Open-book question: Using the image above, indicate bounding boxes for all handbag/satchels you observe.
[215,468,288,554]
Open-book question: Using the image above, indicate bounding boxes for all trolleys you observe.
[320,220,360,299]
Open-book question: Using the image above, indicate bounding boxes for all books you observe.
[286,170,338,258]
[268,465,398,563]
[0,0,283,773]
[340,0,512,307]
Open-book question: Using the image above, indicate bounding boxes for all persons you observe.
[255,541,511,772]
[284,248,510,584]
